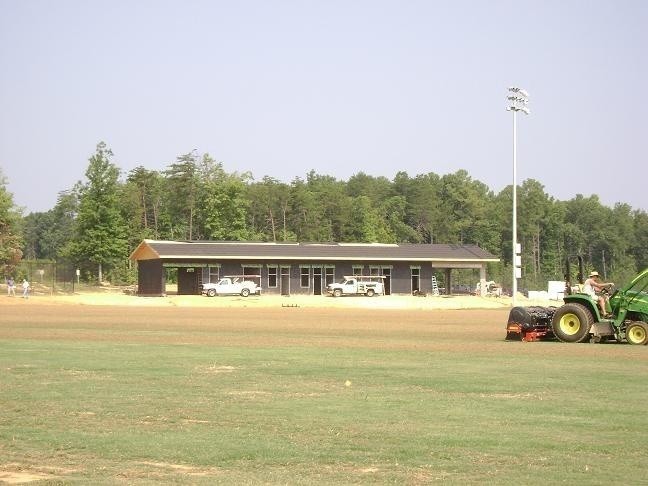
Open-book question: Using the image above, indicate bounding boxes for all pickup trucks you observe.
[201,278,256,297]
[327,278,383,297]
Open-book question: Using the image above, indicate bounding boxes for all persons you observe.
[582,271,614,318]
[7,277,15,297]
[21,279,29,298]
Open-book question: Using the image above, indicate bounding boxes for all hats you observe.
[589,272,599,277]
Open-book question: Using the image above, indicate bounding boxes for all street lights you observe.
[506,86,530,295]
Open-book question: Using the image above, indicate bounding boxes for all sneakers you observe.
[600,313,610,318]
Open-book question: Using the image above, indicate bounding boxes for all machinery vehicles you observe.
[505,254,648,345]
